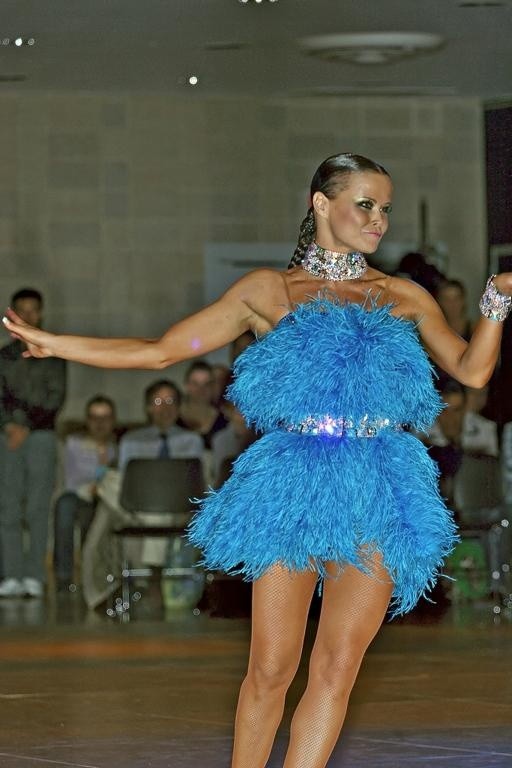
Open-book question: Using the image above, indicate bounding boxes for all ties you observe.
[158,433,170,459]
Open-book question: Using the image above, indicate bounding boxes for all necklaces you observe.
[301,238,370,281]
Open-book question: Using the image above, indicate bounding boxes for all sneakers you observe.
[1,577,44,599]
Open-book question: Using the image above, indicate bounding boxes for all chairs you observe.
[112,457,216,623]
[435,410,512,610]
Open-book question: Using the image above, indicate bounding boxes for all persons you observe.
[0,152,511,768]
[415,279,489,492]
[0,289,67,599]
[58,330,264,613]
